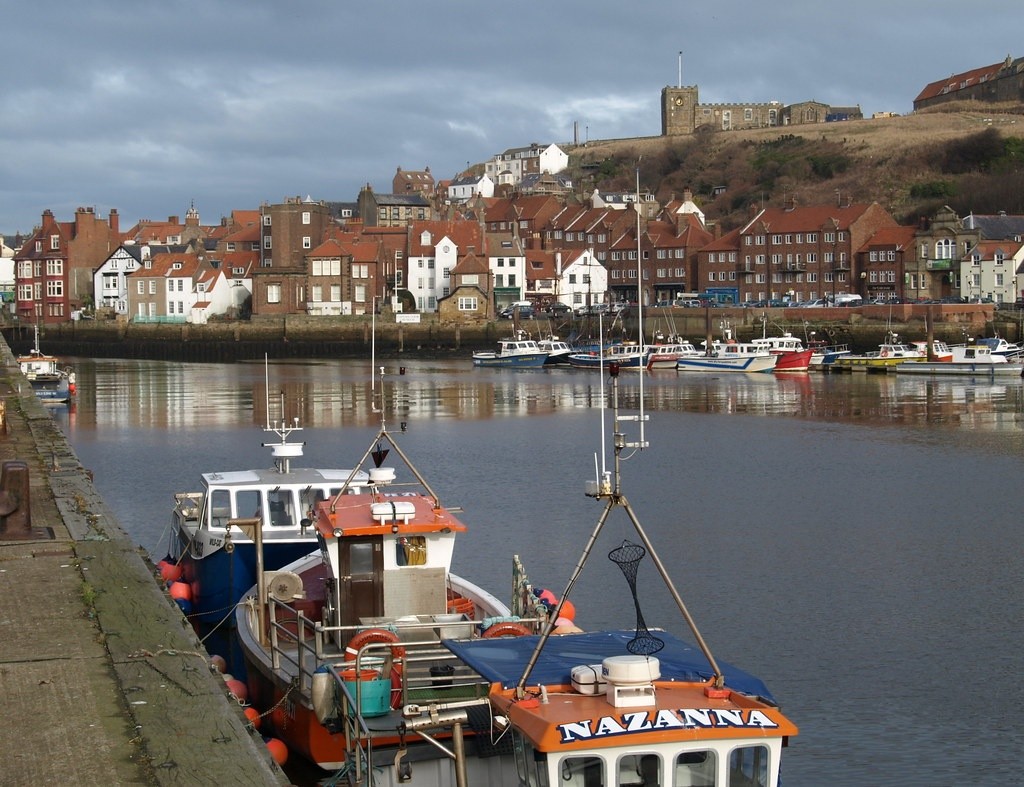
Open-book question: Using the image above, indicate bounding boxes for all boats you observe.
[806,340,853,365]
[538,335,572,363]
[676,310,785,374]
[832,305,1023,376]
[16,325,78,403]
[834,302,928,368]
[750,310,815,371]
[470,330,553,369]
[148,294,798,786]
[568,303,706,369]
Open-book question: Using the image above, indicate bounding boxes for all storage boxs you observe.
[570,665,609,694]
[371,503,415,520]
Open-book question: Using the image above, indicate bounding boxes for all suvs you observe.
[501,305,536,319]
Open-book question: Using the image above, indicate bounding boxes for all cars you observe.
[649,299,719,308]
[544,302,572,313]
[733,293,866,308]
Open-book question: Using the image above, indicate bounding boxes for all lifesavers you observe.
[345,629,407,712]
[481,622,533,637]
[919,350,925,357]
[881,350,888,357]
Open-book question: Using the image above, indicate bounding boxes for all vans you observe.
[497,301,533,317]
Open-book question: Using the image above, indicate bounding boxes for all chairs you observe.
[270,501,286,521]
